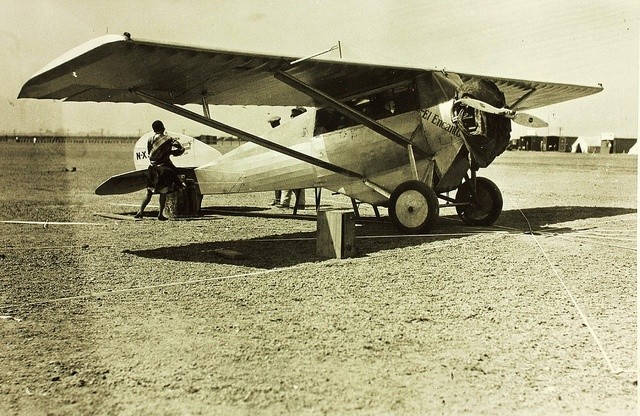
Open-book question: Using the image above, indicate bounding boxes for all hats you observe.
[267,116,281,122]
[292,108,306,113]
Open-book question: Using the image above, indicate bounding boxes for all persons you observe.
[133,120,184,220]
[268,115,282,206]
[282,107,307,209]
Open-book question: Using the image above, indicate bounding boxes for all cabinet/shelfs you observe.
[316,209,355,259]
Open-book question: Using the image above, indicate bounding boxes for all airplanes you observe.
[17,32,604,234]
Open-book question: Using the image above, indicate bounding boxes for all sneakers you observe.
[267,198,280,205]
[298,205,304,209]
[276,203,289,209]
